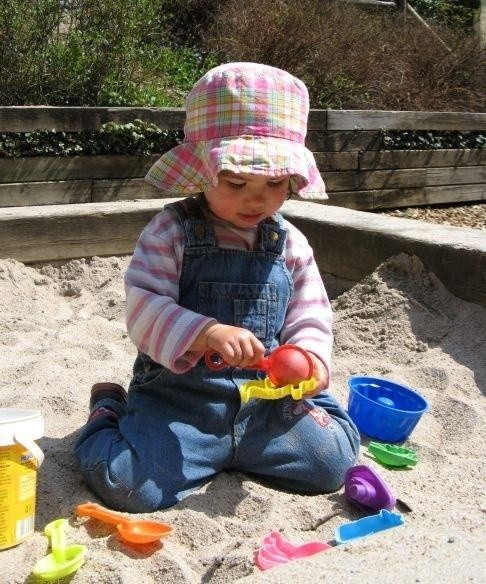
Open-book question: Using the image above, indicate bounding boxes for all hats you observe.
[145,62,329,200]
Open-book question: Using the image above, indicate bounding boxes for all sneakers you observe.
[90,382,127,408]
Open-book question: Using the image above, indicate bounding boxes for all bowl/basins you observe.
[347,376,429,442]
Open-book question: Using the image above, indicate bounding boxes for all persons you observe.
[71,59,366,512]
